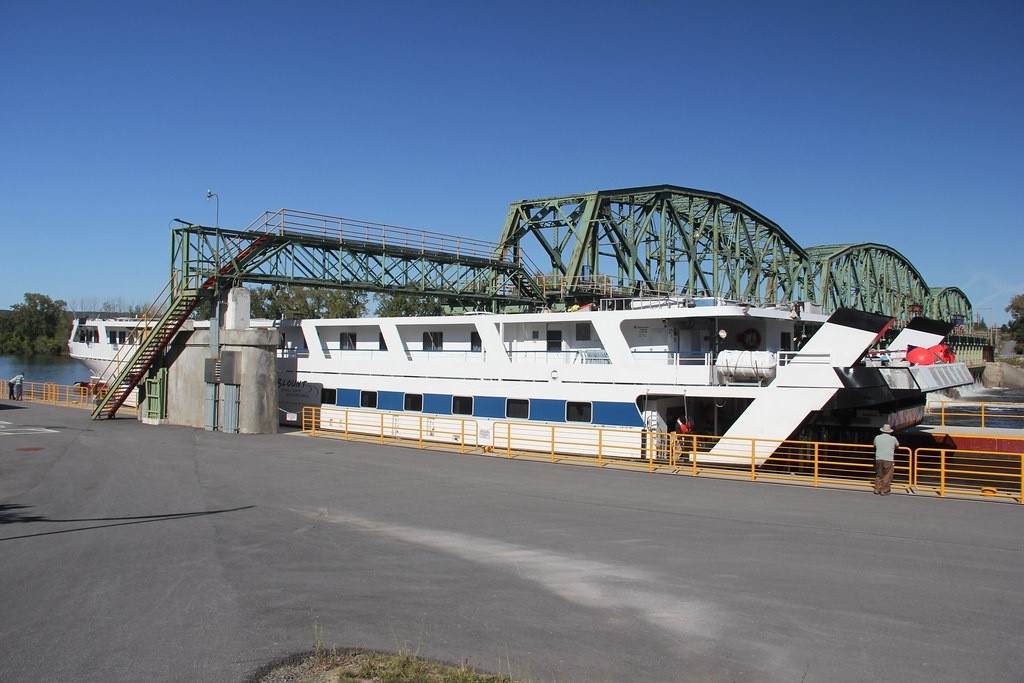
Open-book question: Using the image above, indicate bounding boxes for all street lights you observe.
[204,188,221,289]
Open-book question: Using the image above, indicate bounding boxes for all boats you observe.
[67,295,975,463]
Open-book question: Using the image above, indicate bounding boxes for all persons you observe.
[79,330,85,343]
[8,372,24,401]
[873,424,900,495]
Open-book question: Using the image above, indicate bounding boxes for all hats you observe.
[880,424,894,433]
